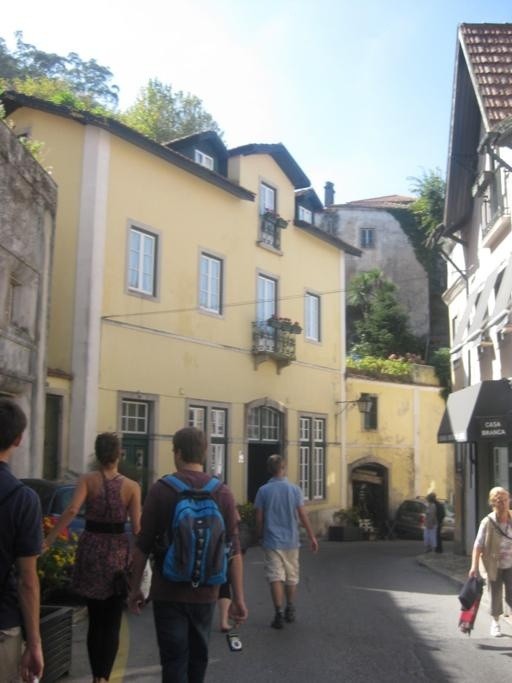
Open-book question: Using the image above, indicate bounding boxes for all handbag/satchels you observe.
[99,466,142,617]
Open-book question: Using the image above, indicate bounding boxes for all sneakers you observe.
[490,622,501,637]
[285,610,296,622]
[271,616,284,629]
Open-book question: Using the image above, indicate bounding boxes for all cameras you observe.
[226,632,243,652]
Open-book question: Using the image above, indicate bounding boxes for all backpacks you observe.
[158,473,225,588]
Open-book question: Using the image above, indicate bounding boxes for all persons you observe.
[421,495,437,553]
[130,426,247,683]
[0,398,45,683]
[218,583,233,633]
[40,431,142,683]
[468,486,512,638]
[251,453,318,631]
[431,493,446,554]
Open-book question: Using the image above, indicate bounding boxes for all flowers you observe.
[293,321,300,328]
[269,313,279,320]
[31,512,79,584]
[280,317,291,325]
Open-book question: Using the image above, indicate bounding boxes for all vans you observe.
[392,494,460,545]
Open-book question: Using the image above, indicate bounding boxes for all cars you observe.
[14,479,142,566]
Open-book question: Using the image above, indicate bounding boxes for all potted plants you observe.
[326,506,362,542]
[236,502,259,544]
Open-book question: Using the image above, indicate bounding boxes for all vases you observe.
[290,327,302,334]
[268,320,282,328]
[280,324,292,330]
